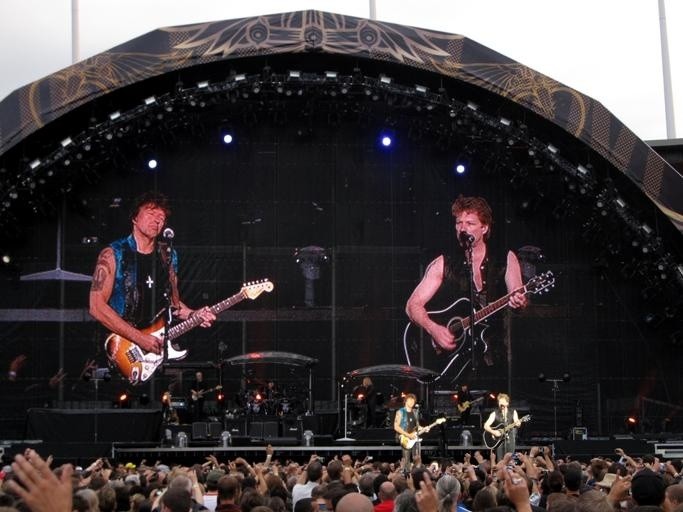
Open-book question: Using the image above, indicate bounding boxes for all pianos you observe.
[344,397,367,409]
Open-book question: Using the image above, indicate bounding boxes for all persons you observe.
[23,366,70,395]
[7,353,27,381]
[403,193,528,390]
[484,392,522,461]
[188,369,213,416]
[88,188,219,355]
[71,358,100,399]
[359,377,377,424]
[161,391,174,422]
[393,393,431,468]
[458,383,476,421]
[0,445,683,512]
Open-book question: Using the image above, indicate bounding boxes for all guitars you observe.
[403,269,557,384]
[400,417,446,450]
[458,397,484,412]
[191,385,223,402]
[105,278,274,388]
[483,414,531,450]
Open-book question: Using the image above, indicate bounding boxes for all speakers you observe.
[554,439,655,466]
[192,421,304,442]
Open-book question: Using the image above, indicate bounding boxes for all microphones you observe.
[459,231,475,242]
[163,228,174,267]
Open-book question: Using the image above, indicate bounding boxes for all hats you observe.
[90,477,106,489]
[162,488,190,512]
[595,473,623,488]
[207,472,224,487]
[126,463,136,469]
[632,471,665,506]
